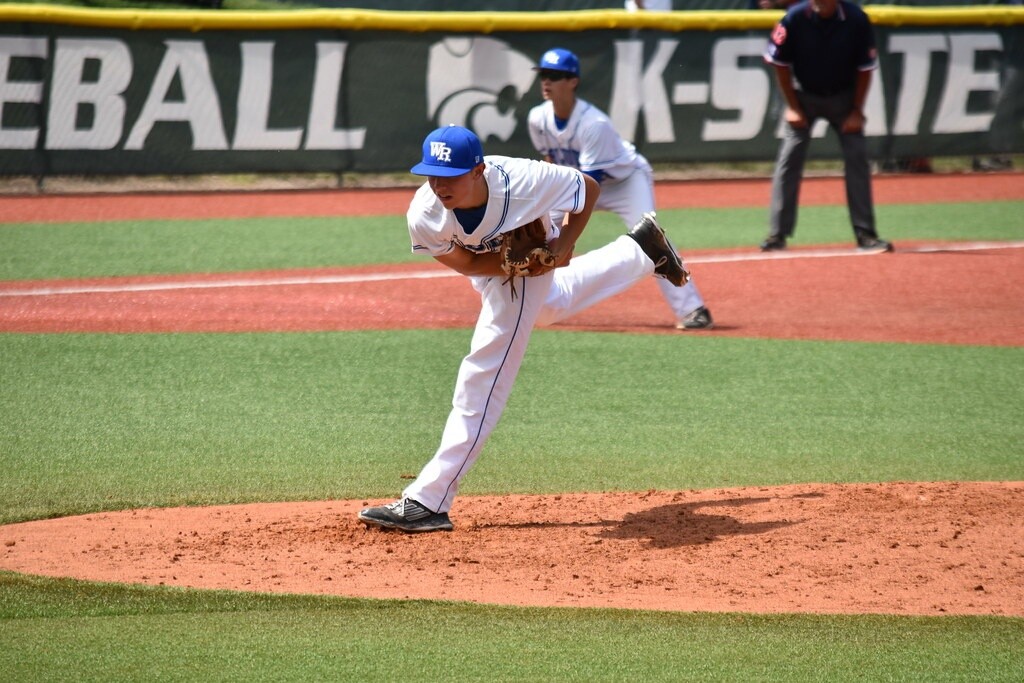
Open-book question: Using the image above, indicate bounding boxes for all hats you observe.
[411,124,484,177]
[531,47,580,77]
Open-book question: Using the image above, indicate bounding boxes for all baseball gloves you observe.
[498,217,555,278]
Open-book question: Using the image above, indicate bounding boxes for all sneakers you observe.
[627,212,690,287]
[855,237,893,253]
[358,497,453,531]
[760,235,786,252]
[677,306,714,330]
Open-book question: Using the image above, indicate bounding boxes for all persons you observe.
[357,123,690,532]
[528,47,715,332]
[972,0,1024,173]
[760,0,894,254]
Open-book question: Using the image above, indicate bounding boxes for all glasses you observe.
[537,71,576,82]
